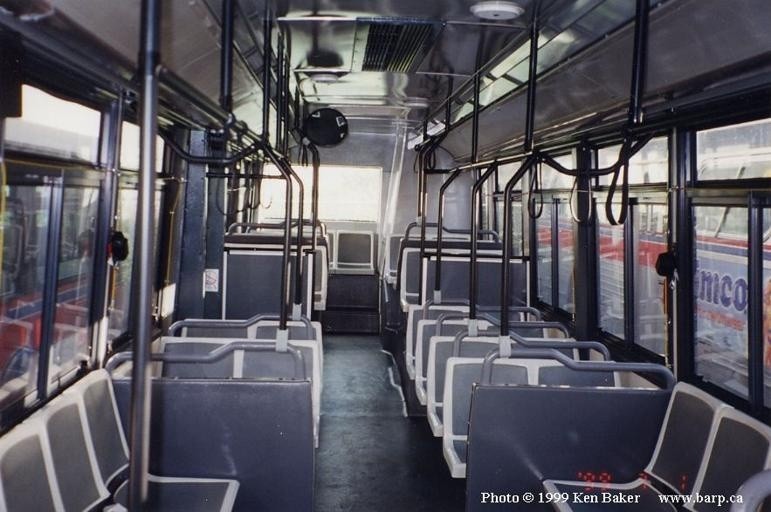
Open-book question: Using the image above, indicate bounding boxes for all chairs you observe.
[0,226,332,512]
[373,234,769,511]
